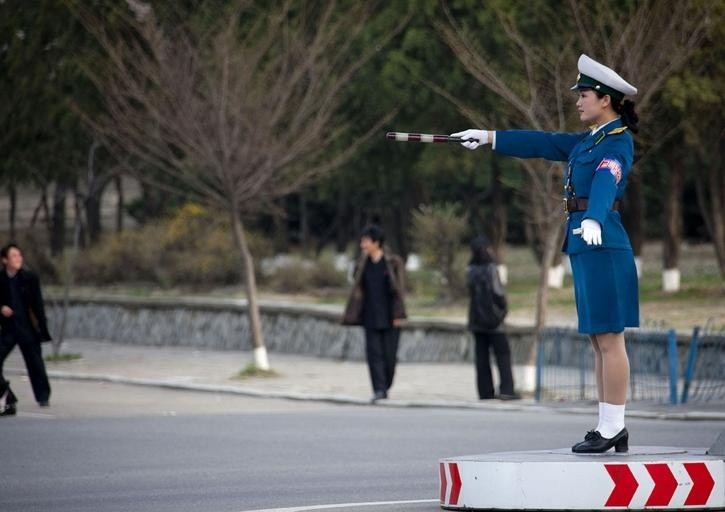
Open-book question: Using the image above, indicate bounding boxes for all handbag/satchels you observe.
[468,272,507,329]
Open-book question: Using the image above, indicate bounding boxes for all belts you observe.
[562,198,620,214]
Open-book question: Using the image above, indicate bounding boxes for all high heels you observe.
[572,426,628,452]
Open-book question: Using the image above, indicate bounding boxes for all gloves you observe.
[581,218,602,246]
[450,129,488,150]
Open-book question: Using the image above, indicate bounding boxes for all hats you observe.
[570,54,638,101]
[469,238,493,251]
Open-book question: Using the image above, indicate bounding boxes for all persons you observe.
[465,235,522,402]
[451,53,640,455]
[342,227,406,403]
[0,244,53,416]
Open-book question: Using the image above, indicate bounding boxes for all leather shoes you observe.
[375,390,386,399]
[480,393,521,400]
[0,404,15,415]
